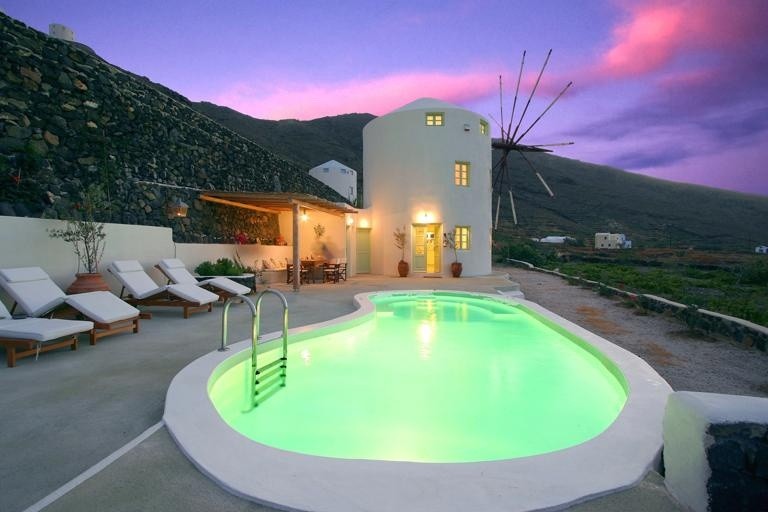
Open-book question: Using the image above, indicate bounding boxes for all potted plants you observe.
[442,230,462,277]
[48,169,114,293]
[394,227,409,277]
[195,257,255,295]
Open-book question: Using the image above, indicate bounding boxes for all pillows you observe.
[263,257,293,269]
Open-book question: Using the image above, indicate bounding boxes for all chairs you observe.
[286,257,348,284]
[0,260,250,369]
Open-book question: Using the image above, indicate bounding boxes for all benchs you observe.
[260,267,307,284]
